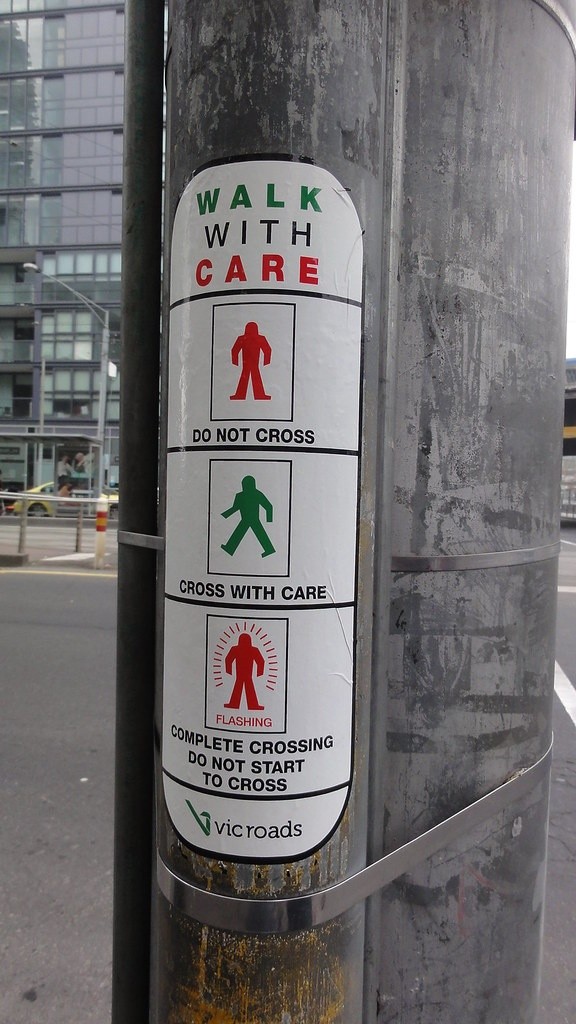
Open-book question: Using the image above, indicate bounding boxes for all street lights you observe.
[22,262,110,519]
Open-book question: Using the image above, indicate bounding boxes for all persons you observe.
[59,482,81,506]
[75,452,96,475]
[58,456,75,476]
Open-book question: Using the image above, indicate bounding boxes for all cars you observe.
[12,480,120,519]
[0,487,20,513]
